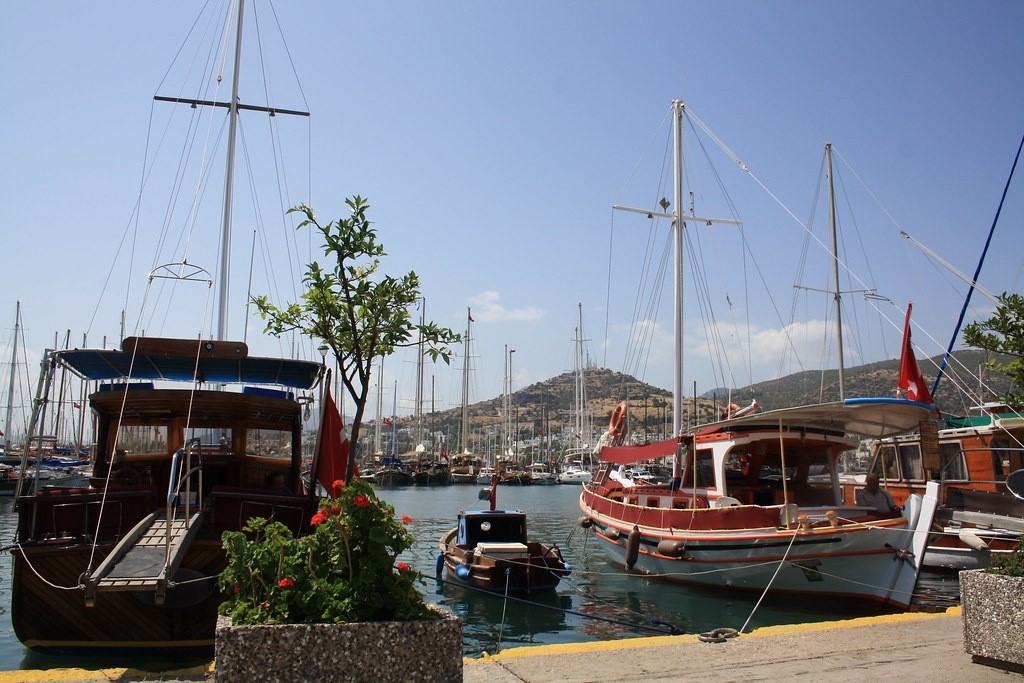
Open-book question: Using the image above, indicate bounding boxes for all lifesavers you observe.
[723,404,742,418]
[608,402,628,436]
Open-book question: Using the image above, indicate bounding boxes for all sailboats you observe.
[1,138,1024,574]
[10,0,352,671]
[576,97,944,611]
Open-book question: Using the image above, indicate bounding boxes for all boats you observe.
[437,472,566,592]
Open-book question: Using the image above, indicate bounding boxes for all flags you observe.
[315,396,360,497]
[898,308,935,404]
[74,402,81,409]
[441,449,448,460]
[384,418,392,427]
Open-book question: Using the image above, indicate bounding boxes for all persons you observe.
[857,473,901,514]
